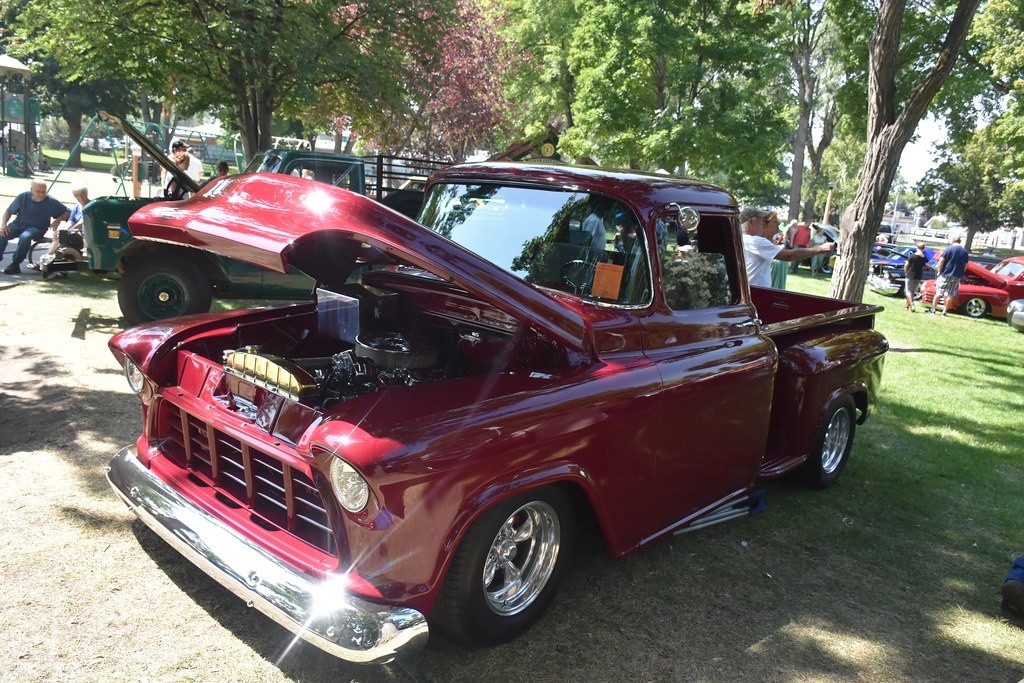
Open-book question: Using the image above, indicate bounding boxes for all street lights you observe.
[822,180,836,226]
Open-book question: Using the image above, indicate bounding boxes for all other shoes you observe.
[911,307,915,312]
[5,263,20,274]
[48,271,68,279]
[925,309,935,314]
[26,263,41,271]
[904,305,909,310]
[942,313,946,316]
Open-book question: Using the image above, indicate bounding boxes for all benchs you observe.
[26,237,54,263]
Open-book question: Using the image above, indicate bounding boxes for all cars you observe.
[812,222,1002,300]
[920,249,1024,319]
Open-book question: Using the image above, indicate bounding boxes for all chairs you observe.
[531,230,608,297]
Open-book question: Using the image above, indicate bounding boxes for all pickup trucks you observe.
[104,163,890,665]
[79,149,455,327]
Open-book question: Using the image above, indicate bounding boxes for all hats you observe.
[172,141,183,148]
[739,208,771,223]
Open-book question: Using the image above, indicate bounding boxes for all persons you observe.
[741,207,835,288]
[161,141,204,199]
[790,218,811,273]
[764,211,788,290]
[924,236,969,317]
[26,185,91,280]
[582,202,606,250]
[302,169,313,180]
[216,161,229,177]
[810,229,827,276]
[783,219,797,245]
[0,178,72,274]
[166,151,191,199]
[981,248,995,269]
[904,242,929,312]
[677,227,697,260]
[876,233,888,250]
[614,218,668,255]
